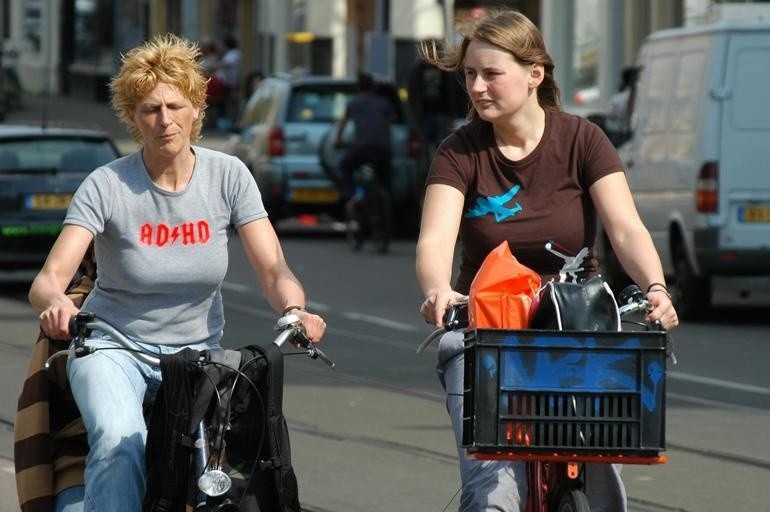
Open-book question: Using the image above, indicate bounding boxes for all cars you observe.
[575,78,602,106]
[0,122,127,286]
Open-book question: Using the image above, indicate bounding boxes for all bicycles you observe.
[332,135,394,257]
[39,311,336,509]
[416,284,680,512]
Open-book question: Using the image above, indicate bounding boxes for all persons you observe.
[415,10,679,512]
[28,36,327,512]
[198,33,245,137]
[408,39,454,208]
[334,70,393,215]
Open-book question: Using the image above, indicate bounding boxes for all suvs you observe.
[219,67,424,236]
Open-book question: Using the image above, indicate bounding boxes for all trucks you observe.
[580,20,770,324]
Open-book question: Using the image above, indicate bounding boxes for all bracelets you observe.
[647,282,672,300]
[282,305,303,316]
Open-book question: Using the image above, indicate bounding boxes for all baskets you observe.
[463,330,666,468]
